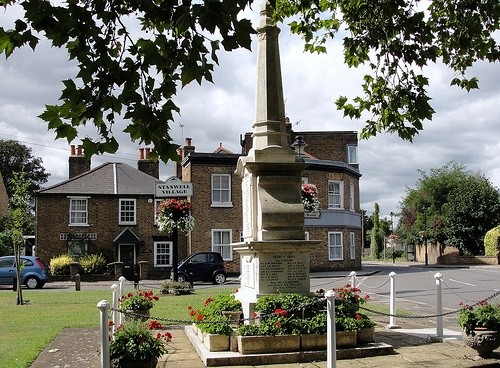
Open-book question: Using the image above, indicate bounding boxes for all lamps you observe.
[292,135,306,156]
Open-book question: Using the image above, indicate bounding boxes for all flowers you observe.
[458,299,500,337]
[186,284,378,336]
[108,319,173,365]
[157,198,196,238]
[117,290,159,312]
[302,182,320,214]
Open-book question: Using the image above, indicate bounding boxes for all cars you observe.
[0,256,52,289]
[170,251,227,285]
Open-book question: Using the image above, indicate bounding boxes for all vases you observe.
[117,357,158,368]
[191,323,376,354]
[462,327,500,361]
[124,310,150,321]
[220,310,243,325]
